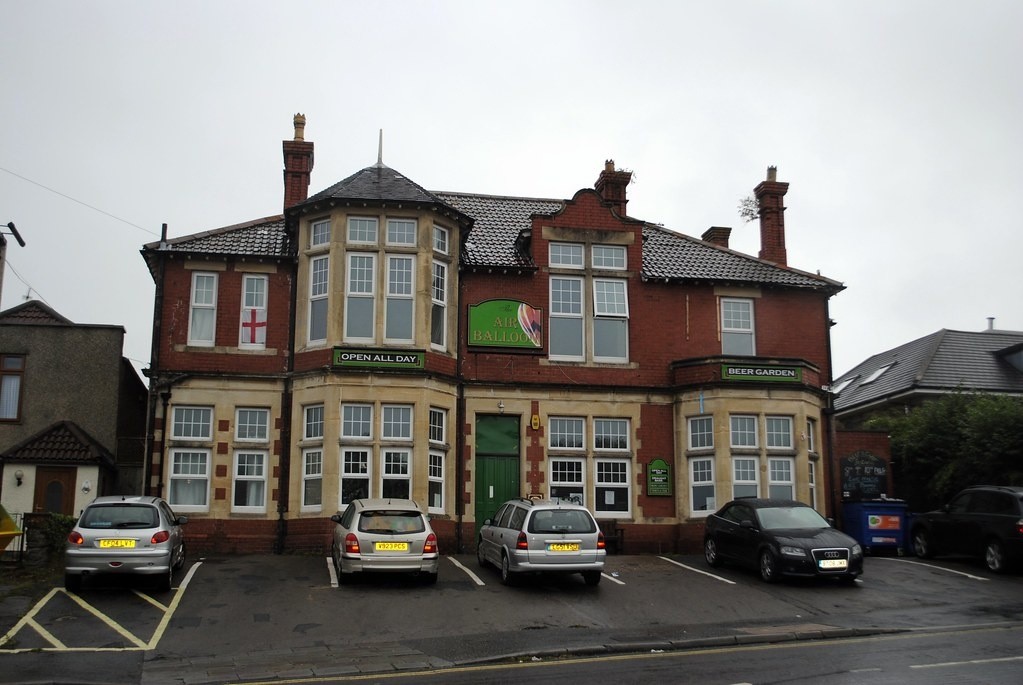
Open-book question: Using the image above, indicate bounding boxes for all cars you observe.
[703,497,864,586]
[328,497,440,588]
[61,494,189,592]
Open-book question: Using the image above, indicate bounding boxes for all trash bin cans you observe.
[840,495,909,557]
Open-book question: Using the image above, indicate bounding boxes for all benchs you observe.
[597,519,626,554]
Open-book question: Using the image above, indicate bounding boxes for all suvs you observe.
[909,483,1023,576]
[475,495,607,587]
